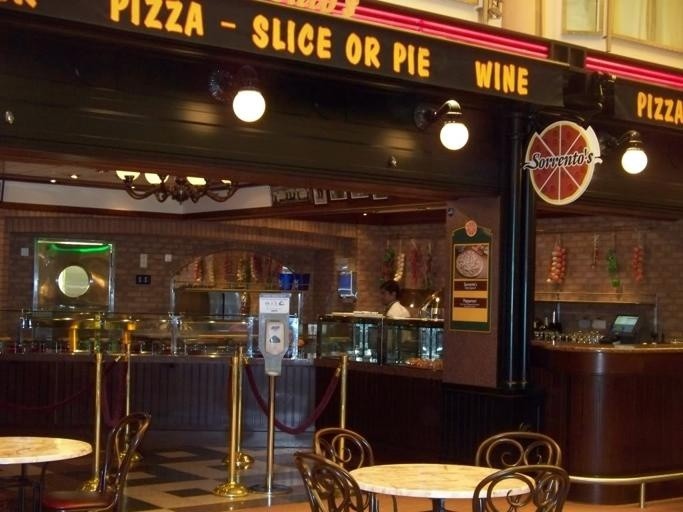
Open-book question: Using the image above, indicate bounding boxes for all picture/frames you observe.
[0,436,93,512]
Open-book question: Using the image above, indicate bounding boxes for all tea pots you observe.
[31,235,115,319]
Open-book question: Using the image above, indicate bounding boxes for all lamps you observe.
[40,410,152,512]
[293,428,569,512]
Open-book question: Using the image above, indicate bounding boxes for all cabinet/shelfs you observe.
[533,309,562,338]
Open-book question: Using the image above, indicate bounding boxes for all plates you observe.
[0,306,299,360]
[315,315,444,378]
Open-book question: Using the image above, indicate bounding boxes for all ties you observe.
[552,302,561,324]
[608,313,643,338]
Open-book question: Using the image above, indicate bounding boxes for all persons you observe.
[378,280,411,353]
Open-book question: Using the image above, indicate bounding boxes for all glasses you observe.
[413,100,469,152]
[599,130,648,174]
[53,261,91,299]
[210,64,267,123]
[115,170,238,203]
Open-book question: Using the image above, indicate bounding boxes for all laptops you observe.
[405,358,443,371]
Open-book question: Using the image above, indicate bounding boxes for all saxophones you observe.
[393,253,405,281]
[547,246,567,284]
[629,244,648,282]
